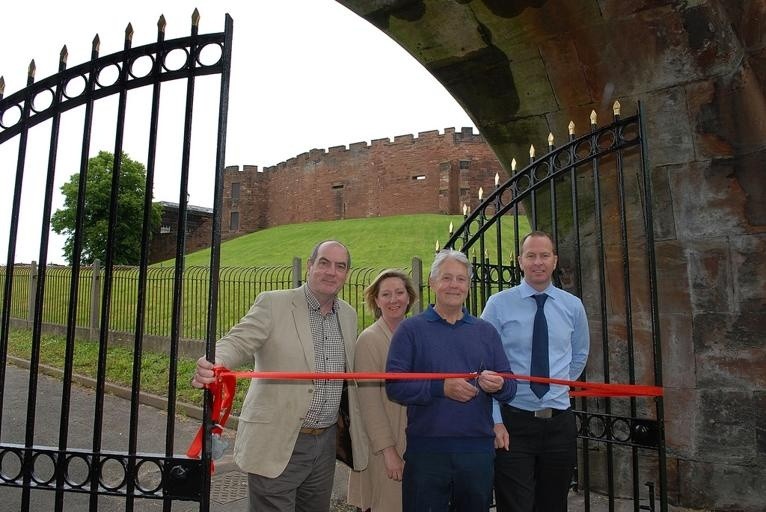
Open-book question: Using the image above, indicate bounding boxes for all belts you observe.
[298,425,329,437]
[500,401,565,420]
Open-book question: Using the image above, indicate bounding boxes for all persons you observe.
[192,241,369,512]
[347,269,417,512]
[480,232,590,512]
[386,248,516,512]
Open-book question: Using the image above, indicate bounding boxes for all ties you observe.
[529,291,553,401]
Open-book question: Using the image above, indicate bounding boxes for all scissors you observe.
[467,360,486,400]
